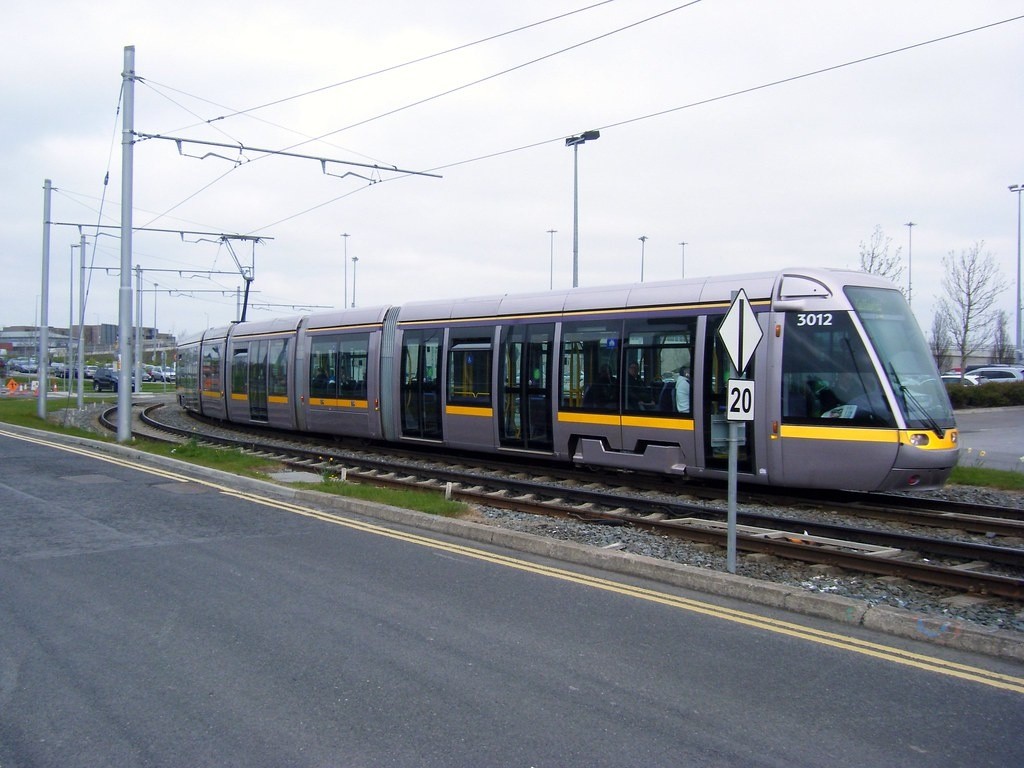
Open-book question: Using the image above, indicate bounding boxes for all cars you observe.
[512,367,716,391]
[0,355,176,383]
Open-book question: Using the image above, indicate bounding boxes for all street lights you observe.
[340,232,350,308]
[351,257,359,308]
[1008,184,1024,366]
[546,229,557,290]
[153,282,159,364]
[638,236,648,282]
[904,221,917,307]
[678,241,689,279]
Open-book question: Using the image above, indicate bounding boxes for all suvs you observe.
[942,363,1024,388]
[93,367,141,393]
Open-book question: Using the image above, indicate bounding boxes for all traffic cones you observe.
[51,381,59,392]
[33,386,39,396]
[19,382,28,394]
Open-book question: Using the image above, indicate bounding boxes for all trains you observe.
[175,268,961,496]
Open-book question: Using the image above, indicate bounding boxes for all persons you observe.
[329,367,349,399]
[627,360,654,412]
[675,365,691,413]
[834,366,868,408]
[311,367,328,398]
[592,364,617,409]
[273,374,287,396]
